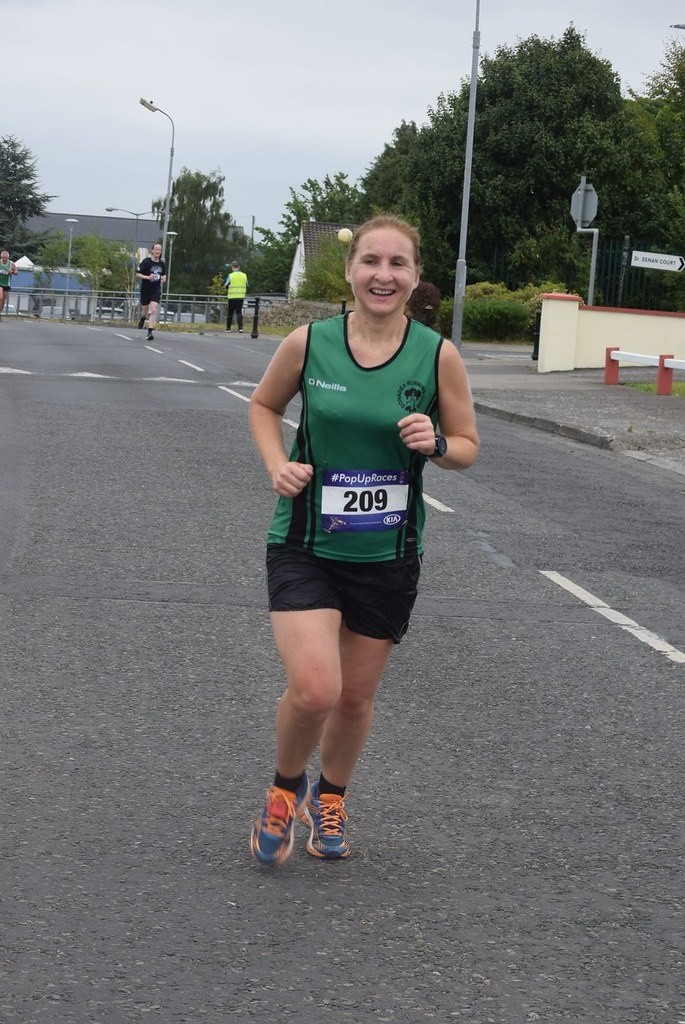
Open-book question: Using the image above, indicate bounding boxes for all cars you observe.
[1,305,178,327]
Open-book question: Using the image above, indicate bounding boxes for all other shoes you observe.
[225,329,231,332]
[238,328,243,333]
[146,335,154,340]
[138,319,146,329]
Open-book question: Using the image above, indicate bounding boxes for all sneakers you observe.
[248,772,311,867]
[299,781,351,858]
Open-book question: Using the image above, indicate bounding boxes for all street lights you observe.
[105,205,168,326]
[136,98,177,327]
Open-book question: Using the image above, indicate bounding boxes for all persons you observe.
[250,218,480,866]
[0,251,19,323]
[135,244,166,340]
[225,261,249,333]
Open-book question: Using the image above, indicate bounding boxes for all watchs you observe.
[426,433,448,459]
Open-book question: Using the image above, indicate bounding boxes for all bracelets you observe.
[12,271,14,274]
